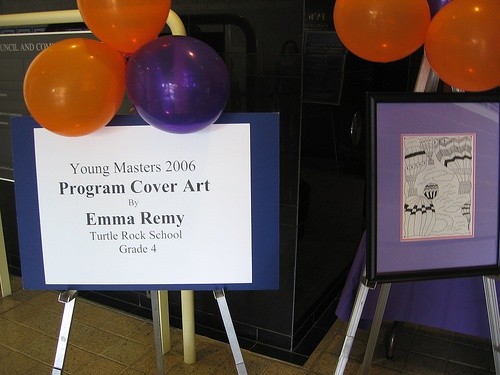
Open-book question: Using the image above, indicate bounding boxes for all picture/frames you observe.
[363,90,500,286]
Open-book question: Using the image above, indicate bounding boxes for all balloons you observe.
[77,0,172,55]
[23,38,126,137]
[425,0,500,92]
[126,35,231,134]
[334,0,430,63]
